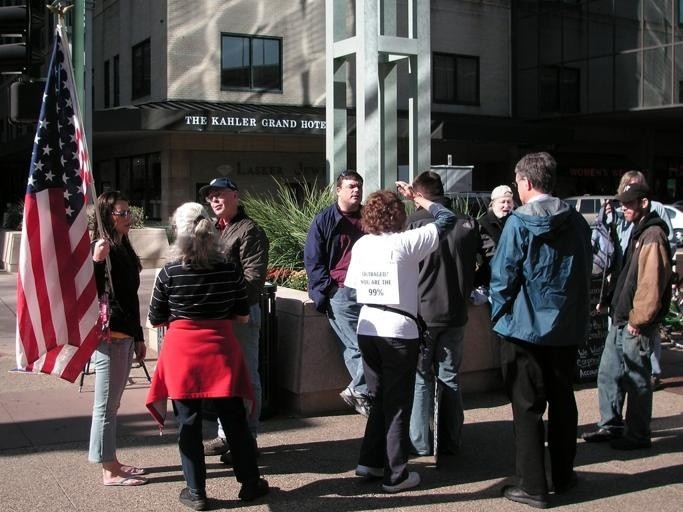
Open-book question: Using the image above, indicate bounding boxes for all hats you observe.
[488,185,513,206]
[199,177,239,197]
[611,183,652,203]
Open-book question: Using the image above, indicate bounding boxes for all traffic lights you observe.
[0,0,43,76]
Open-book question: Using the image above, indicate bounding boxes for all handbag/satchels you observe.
[416,313,427,334]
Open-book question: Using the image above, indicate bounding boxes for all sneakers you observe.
[356,465,385,477]
[552,471,577,492]
[502,485,550,509]
[608,437,653,450]
[220,452,232,463]
[382,472,421,492]
[340,385,371,418]
[204,438,229,455]
[239,479,268,500]
[582,428,623,443]
[180,488,208,510]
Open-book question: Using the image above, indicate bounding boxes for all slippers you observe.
[102,465,148,485]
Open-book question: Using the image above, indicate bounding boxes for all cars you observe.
[562,193,616,231]
[663,204,683,247]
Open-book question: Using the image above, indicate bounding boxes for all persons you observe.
[581,181,672,450]
[303,168,373,420]
[143,201,269,512]
[343,180,459,495]
[88,187,150,487]
[487,150,593,507]
[475,185,516,318]
[402,169,492,459]
[198,176,269,463]
[604,169,678,392]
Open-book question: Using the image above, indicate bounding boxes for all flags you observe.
[8,27,105,386]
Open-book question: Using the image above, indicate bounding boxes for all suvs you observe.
[444,191,519,221]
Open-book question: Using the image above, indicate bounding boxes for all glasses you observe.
[205,191,232,202]
[111,209,131,215]
